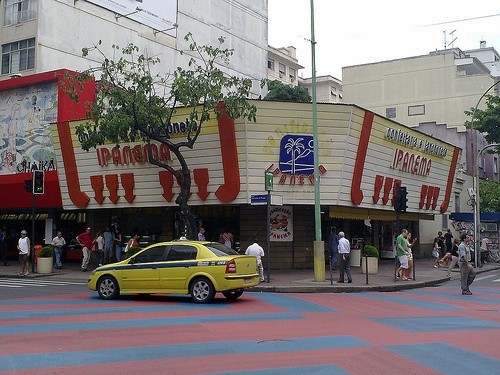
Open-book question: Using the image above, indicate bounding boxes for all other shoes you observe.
[261,280,266,282]
[433,265,437,267]
[17,273,22,274]
[85,268,89,270]
[462,289,472,295]
[54,267,57,269]
[24,273,29,275]
[59,266,62,269]
[82,267,85,272]
[446,274,451,277]
[336,280,342,283]
[439,260,443,262]
[99,264,102,266]
[408,276,413,279]
[439,264,442,267]
[347,280,352,283]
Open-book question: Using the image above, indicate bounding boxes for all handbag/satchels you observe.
[432,250,437,256]
[125,239,134,252]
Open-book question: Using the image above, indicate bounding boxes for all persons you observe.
[481,236,489,263]
[337,231,353,283]
[20,157,55,173]
[468,234,475,265]
[50,231,66,269]
[0,228,10,266]
[17,230,31,276]
[113,228,122,262]
[96,232,105,267]
[432,229,461,278]
[70,236,77,243]
[222,228,233,249]
[127,233,138,263]
[198,226,206,241]
[457,232,476,295]
[393,229,417,281]
[76,226,95,271]
[244,238,266,282]
[328,228,339,274]
[31,91,42,112]
[103,225,114,263]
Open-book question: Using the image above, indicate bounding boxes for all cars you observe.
[88,237,260,303]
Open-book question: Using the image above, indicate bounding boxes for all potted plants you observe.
[361,245,379,274]
[37,246,54,274]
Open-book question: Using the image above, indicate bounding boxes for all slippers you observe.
[396,274,399,279]
[403,278,410,281]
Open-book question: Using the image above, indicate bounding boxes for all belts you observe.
[462,261,471,262]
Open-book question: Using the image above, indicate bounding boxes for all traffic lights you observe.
[397,186,409,214]
[32,170,44,194]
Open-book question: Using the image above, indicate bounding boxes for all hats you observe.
[87,227,91,230]
